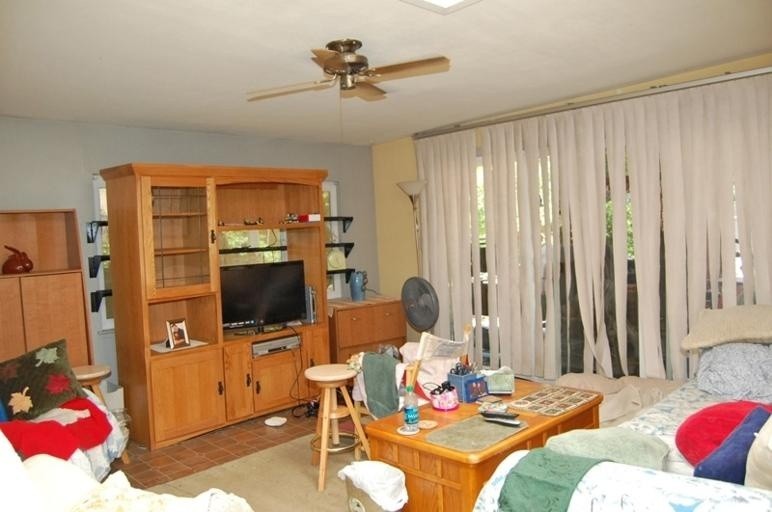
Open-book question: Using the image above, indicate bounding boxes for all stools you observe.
[304,363,370,493]
[71,365,130,465]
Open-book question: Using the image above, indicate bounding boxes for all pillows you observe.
[501,448,602,512]
[673,399,772,485]
[544,427,671,472]
[0,338,87,424]
[679,306,772,350]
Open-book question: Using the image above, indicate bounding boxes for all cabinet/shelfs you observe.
[0,207,94,369]
[88,215,112,313]
[324,215,355,284]
[328,305,407,385]
[100,162,331,451]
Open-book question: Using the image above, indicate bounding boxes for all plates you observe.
[264,415,288,427]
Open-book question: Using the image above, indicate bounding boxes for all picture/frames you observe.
[166,318,190,349]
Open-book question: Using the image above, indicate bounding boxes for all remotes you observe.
[481,410,520,419]
[484,417,521,426]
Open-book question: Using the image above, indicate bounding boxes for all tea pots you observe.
[2,244,34,275]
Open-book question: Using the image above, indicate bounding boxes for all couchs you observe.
[471,342,772,512]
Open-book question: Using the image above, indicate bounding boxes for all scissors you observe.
[450,362,468,376]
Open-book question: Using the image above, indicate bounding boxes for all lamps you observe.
[396,181,428,277]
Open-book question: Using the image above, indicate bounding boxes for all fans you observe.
[246,39,451,105]
[401,280,439,335]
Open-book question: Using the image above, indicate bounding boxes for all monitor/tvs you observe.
[219,259,308,336]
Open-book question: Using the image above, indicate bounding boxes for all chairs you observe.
[353,325,470,462]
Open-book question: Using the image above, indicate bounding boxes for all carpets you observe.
[141,431,386,512]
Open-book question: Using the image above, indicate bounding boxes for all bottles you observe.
[404,385,420,431]
